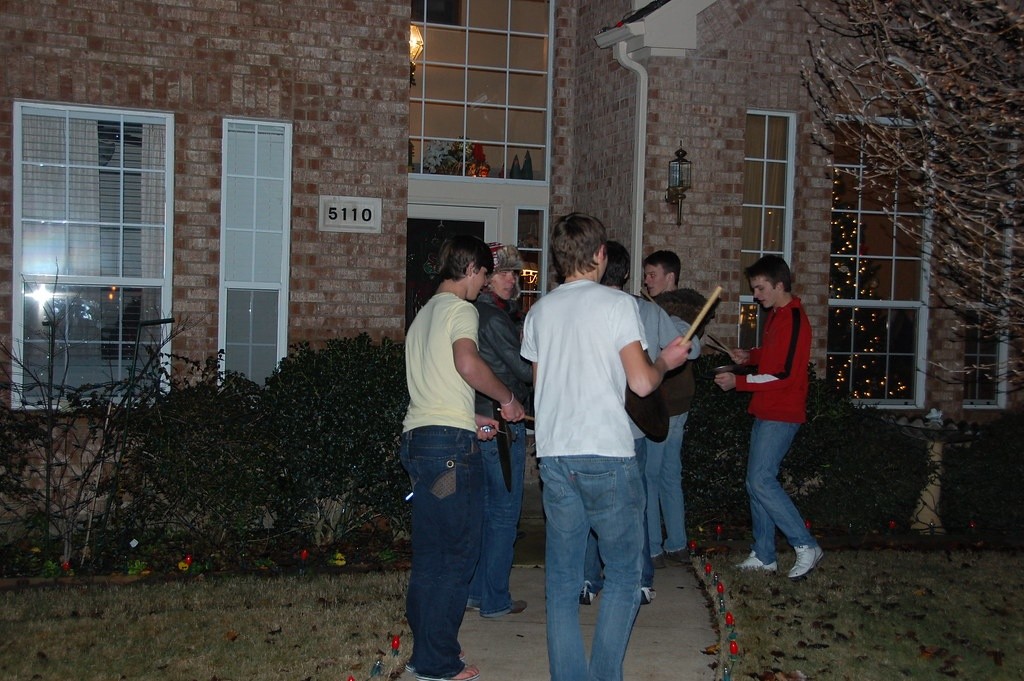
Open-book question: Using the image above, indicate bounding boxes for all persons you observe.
[519,211,717,681]
[463,241,529,619]
[399,241,526,681]
[713,253,825,579]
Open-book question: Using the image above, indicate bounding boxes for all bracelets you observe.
[500,391,515,408]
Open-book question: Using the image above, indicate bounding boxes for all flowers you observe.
[424,137,489,177]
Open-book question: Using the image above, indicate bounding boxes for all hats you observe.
[485,242,522,301]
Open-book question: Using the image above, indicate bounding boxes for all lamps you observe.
[662,138,693,228]
[410,25,422,91]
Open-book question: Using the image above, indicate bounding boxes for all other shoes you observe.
[506,599,528,617]
[663,550,689,564]
[640,587,656,605]
[465,603,479,612]
[651,554,664,569]
[579,581,596,605]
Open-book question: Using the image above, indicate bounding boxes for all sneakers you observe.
[787,546,823,578]
[734,550,777,573]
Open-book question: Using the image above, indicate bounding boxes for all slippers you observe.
[415,665,480,681]
[405,651,466,674]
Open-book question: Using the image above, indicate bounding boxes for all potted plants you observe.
[408,140,416,173]
